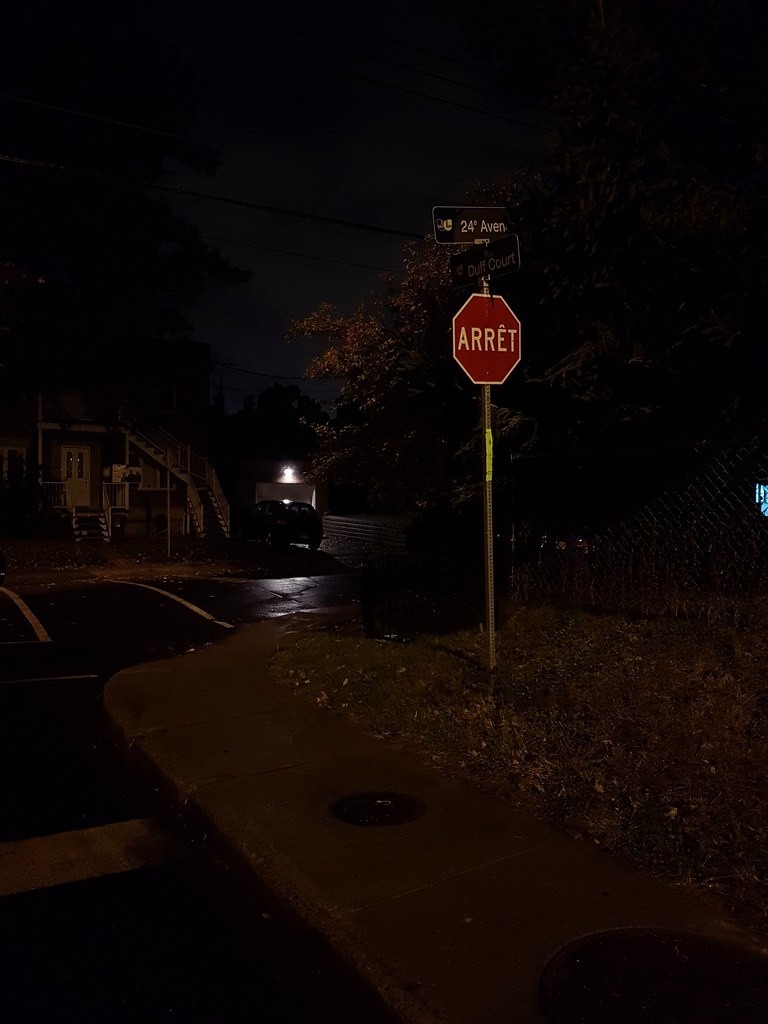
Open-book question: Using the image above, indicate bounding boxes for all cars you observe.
[240,500,324,550]
